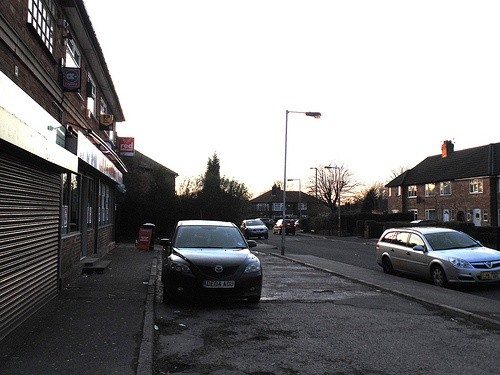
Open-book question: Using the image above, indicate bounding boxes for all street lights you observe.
[287,178,302,231]
[281,110,322,255]
[324,166,341,238]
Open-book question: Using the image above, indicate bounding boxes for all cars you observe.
[258,217,274,229]
[376,226,500,287]
[161,220,263,304]
[274,218,295,234]
[241,219,269,238]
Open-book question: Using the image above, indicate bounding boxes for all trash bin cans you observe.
[142,223,155,249]
